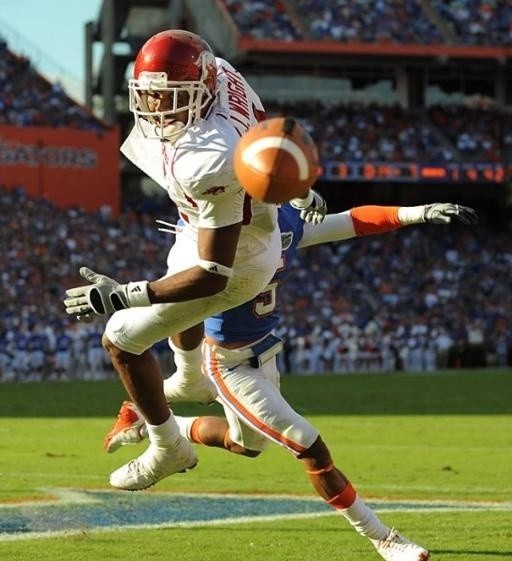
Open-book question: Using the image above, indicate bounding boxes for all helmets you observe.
[128,29,216,140]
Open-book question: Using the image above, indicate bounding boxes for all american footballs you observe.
[233,117,319,203]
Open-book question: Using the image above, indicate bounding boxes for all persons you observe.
[0,40,103,127]
[0,184,177,381]
[105,197,478,561]
[223,1,512,47]
[64,27,329,492]
[264,100,509,162]
[279,226,510,371]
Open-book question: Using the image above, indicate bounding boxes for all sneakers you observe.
[110,441,197,491]
[105,402,146,453]
[375,530,430,561]
[162,375,217,406]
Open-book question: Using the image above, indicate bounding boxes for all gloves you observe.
[64,267,151,321]
[290,190,327,225]
[425,203,479,224]
